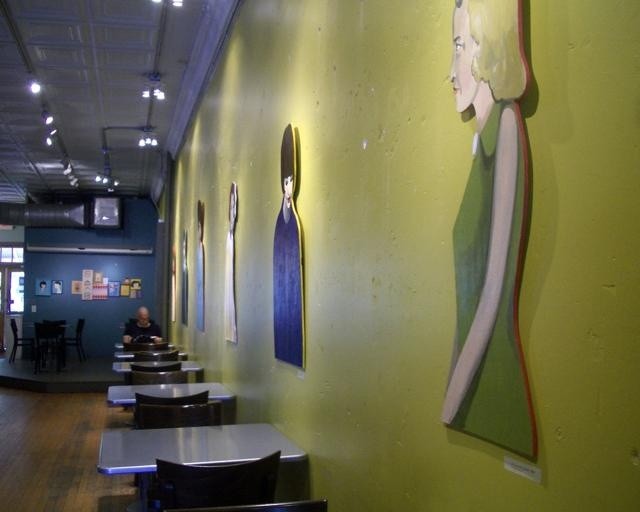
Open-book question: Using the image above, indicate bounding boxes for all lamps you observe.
[25,72,166,193]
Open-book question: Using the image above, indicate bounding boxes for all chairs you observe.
[96,341,328,511]
[8,317,86,376]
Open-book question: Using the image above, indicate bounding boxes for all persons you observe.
[122,306,164,344]
